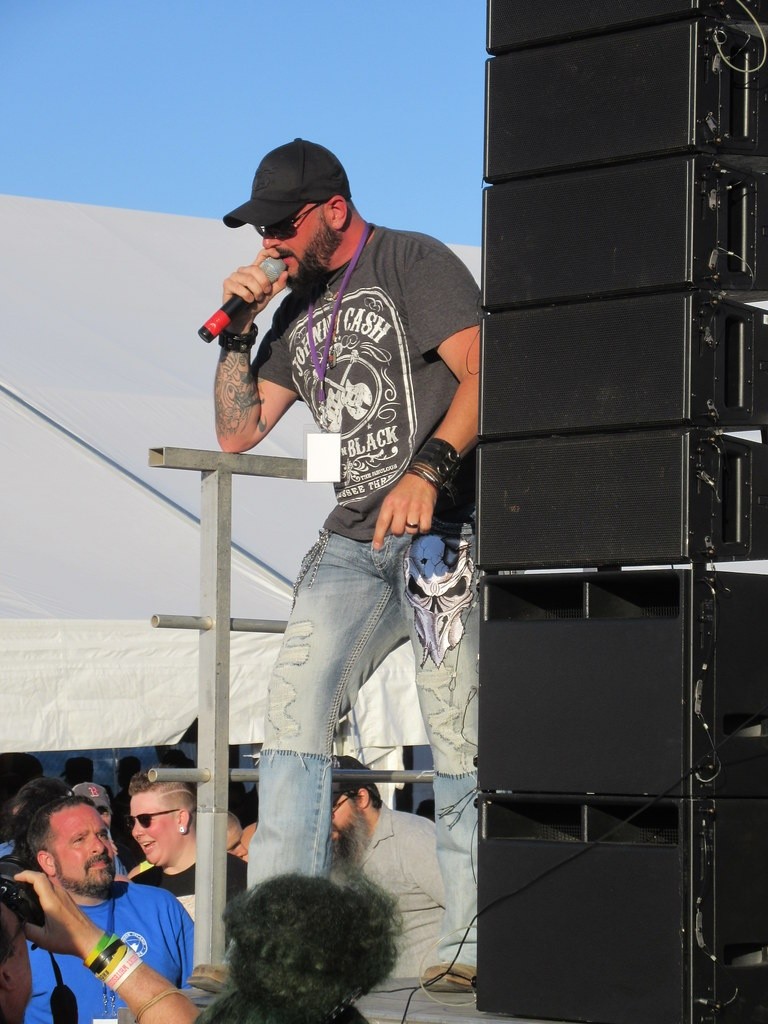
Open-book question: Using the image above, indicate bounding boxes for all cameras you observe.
[0,854,45,928]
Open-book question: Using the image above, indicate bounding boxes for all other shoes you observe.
[423,963,476,993]
[187,967,234,993]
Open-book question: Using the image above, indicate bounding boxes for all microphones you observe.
[197,257,287,344]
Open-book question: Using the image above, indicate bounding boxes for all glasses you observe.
[123,808,181,831]
[255,200,324,241]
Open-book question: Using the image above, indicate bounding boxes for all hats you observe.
[69,784,113,816]
[222,137,351,227]
[328,757,372,806]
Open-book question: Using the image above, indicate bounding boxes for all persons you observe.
[192,876,394,1024]
[172,139,481,993]
[329,755,446,975]
[1,870,201,1023]
[24,796,194,1024]
[68,782,125,877]
[0,776,67,857]
[128,812,257,879]
[129,765,247,931]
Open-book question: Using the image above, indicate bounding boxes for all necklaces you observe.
[319,297,338,368]
[320,261,349,300]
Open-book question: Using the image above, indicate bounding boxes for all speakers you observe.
[481,1,768,1023]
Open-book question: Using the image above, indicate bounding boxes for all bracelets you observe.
[408,461,443,492]
[219,323,258,352]
[419,439,460,477]
[83,933,143,992]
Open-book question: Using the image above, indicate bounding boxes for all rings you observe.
[407,522,419,528]
